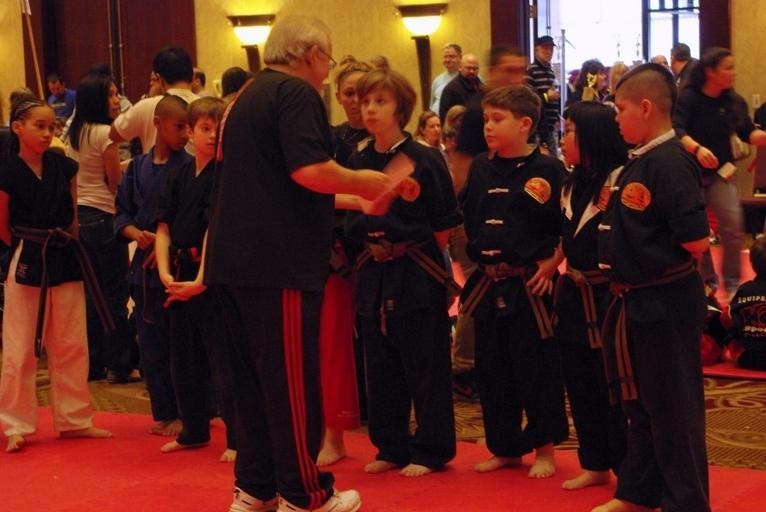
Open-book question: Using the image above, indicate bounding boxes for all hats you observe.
[535,36,555,47]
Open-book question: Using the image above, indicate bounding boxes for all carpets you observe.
[0,403,765,512]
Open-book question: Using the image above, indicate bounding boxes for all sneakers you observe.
[230,486,279,512]
[277,489,362,511]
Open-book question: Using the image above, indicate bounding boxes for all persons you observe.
[566,44,766,373]
[48,46,252,462]
[0,89,113,452]
[559,104,626,492]
[590,60,723,512]
[314,37,573,478]
[215,16,363,512]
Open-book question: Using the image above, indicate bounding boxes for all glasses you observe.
[318,49,338,70]
